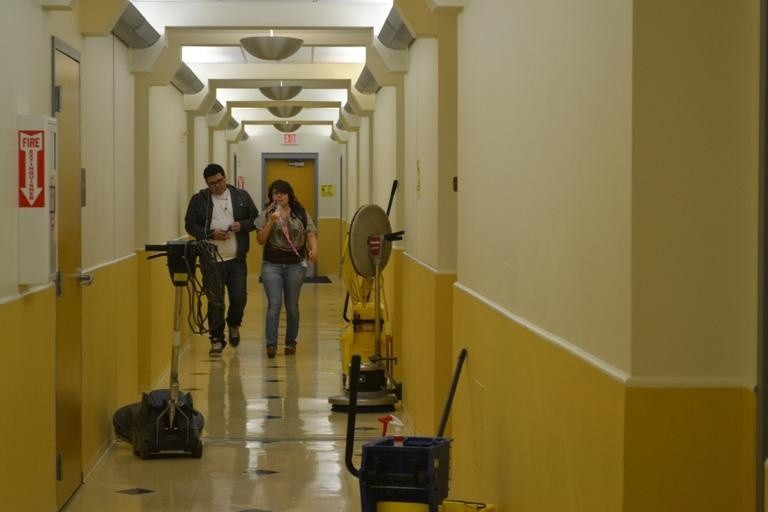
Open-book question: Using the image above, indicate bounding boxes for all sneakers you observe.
[284,344,296,355]
[209,336,228,358]
[228,325,240,347]
[267,344,276,358]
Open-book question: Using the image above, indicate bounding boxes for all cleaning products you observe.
[377,413,405,447]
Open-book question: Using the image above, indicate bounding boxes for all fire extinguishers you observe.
[238,176,244,190]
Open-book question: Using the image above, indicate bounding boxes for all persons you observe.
[184,162,261,358]
[254,179,320,357]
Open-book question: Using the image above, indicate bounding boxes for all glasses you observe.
[208,177,225,188]
